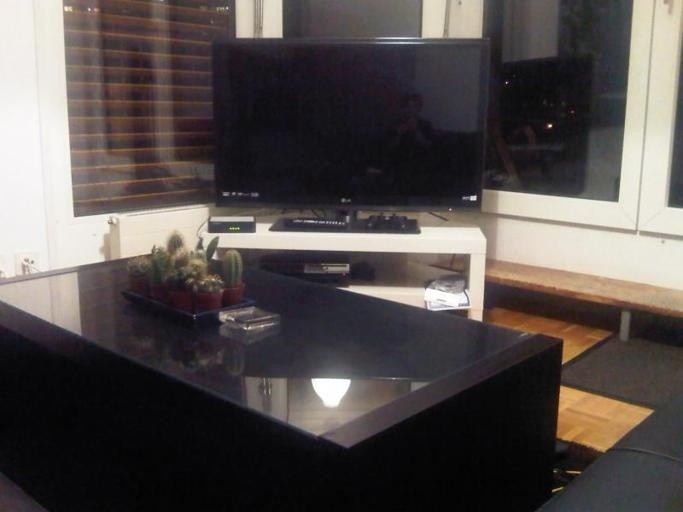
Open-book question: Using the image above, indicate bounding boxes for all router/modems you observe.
[208,215,256,233]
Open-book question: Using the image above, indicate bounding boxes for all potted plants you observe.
[120,229,246,311]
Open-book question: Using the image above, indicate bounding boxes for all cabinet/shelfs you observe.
[200,219,486,323]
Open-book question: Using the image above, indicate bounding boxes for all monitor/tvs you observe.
[210,37,492,235]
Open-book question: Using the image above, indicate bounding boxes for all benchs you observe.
[485,258,683,344]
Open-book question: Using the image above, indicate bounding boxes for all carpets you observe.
[532,327,682,411]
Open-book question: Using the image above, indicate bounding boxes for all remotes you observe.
[288,217,348,229]
